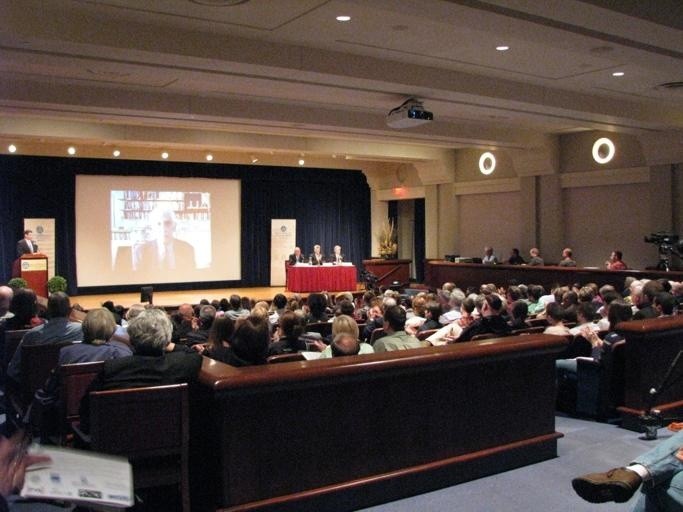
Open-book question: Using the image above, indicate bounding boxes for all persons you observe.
[528,247,543,266]
[0,436,52,512]
[1,284,307,448]
[481,245,498,264]
[16,230,38,256]
[560,248,576,267]
[309,243,325,265]
[300,277,681,379]
[289,247,301,263]
[572,429,683,510]
[327,245,346,262]
[135,208,194,269]
[604,251,625,270]
[508,248,526,265]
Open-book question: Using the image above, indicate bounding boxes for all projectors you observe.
[385,109,433,129]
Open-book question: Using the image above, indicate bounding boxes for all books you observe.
[20,445,133,508]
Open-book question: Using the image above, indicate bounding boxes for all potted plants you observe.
[377,216,397,259]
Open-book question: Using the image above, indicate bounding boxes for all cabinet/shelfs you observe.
[119,191,210,223]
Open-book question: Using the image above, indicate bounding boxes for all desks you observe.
[288,265,357,292]
[363,257,412,286]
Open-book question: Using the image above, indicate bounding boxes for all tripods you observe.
[656,247,675,271]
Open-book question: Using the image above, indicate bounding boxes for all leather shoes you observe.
[572,466,641,503]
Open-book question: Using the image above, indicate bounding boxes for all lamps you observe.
[250,155,258,163]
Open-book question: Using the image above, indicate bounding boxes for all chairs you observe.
[369,327,386,343]
[5,343,51,424]
[285,259,291,288]
[470,333,496,339]
[305,322,332,334]
[418,329,437,339]
[357,323,366,335]
[268,352,304,361]
[529,318,549,326]
[1,330,31,410]
[41,360,104,445]
[267,354,303,361]
[72,384,191,510]
[558,338,625,418]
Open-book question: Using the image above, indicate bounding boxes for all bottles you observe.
[308,257,312,266]
